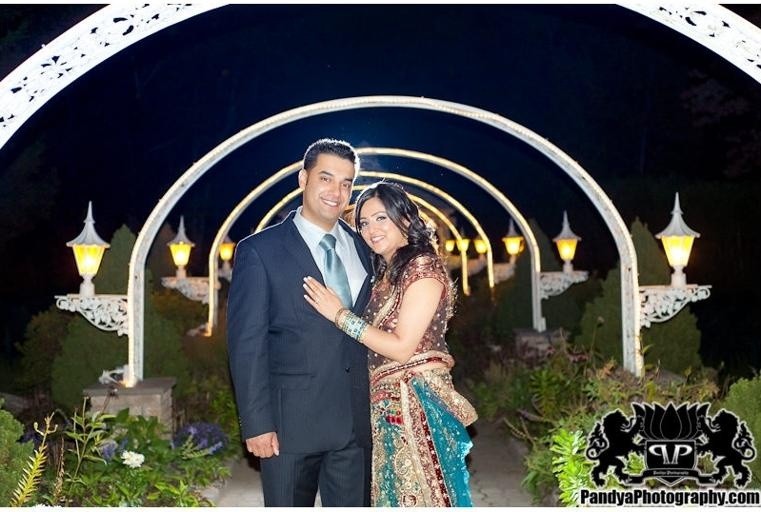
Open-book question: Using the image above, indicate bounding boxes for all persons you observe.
[302,179,479,508]
[224,138,383,506]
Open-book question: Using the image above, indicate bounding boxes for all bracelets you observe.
[335,306,369,343]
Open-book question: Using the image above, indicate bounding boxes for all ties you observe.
[319,235,353,311]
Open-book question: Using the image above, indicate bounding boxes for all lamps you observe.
[166,217,195,272]
[455,219,528,256]
[552,211,582,272]
[66,201,112,296]
[215,234,236,269]
[654,191,702,287]
